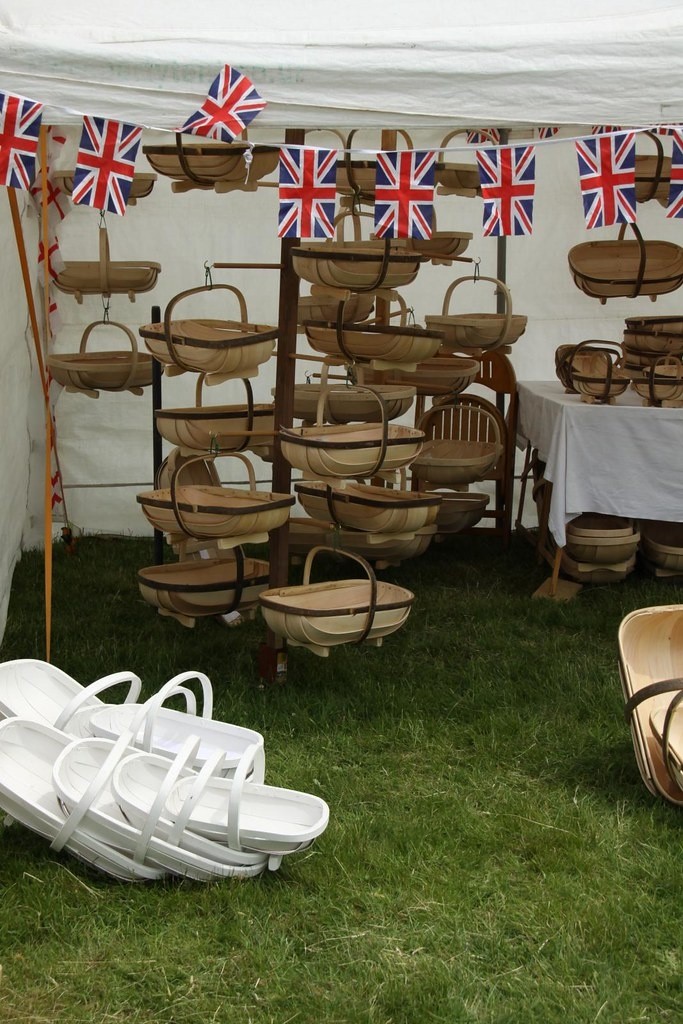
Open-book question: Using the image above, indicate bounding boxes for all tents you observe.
[0,4,682,643]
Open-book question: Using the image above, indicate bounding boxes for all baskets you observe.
[632,131,674,207]
[532,446,683,582]
[136,212,527,658]
[0,659,330,882]
[52,226,161,303]
[616,603,682,805]
[142,128,499,206]
[52,169,157,205]
[555,316,683,409]
[567,220,683,303]
[47,321,164,398]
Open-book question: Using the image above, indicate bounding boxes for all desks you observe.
[514,378,683,595]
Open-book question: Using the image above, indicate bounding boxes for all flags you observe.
[666,127,683,220]
[33,125,70,523]
[0,90,44,193]
[591,126,620,134]
[277,147,338,238]
[71,115,143,219]
[179,64,267,144]
[467,129,502,144]
[477,146,535,235]
[373,152,434,240]
[576,133,637,229]
